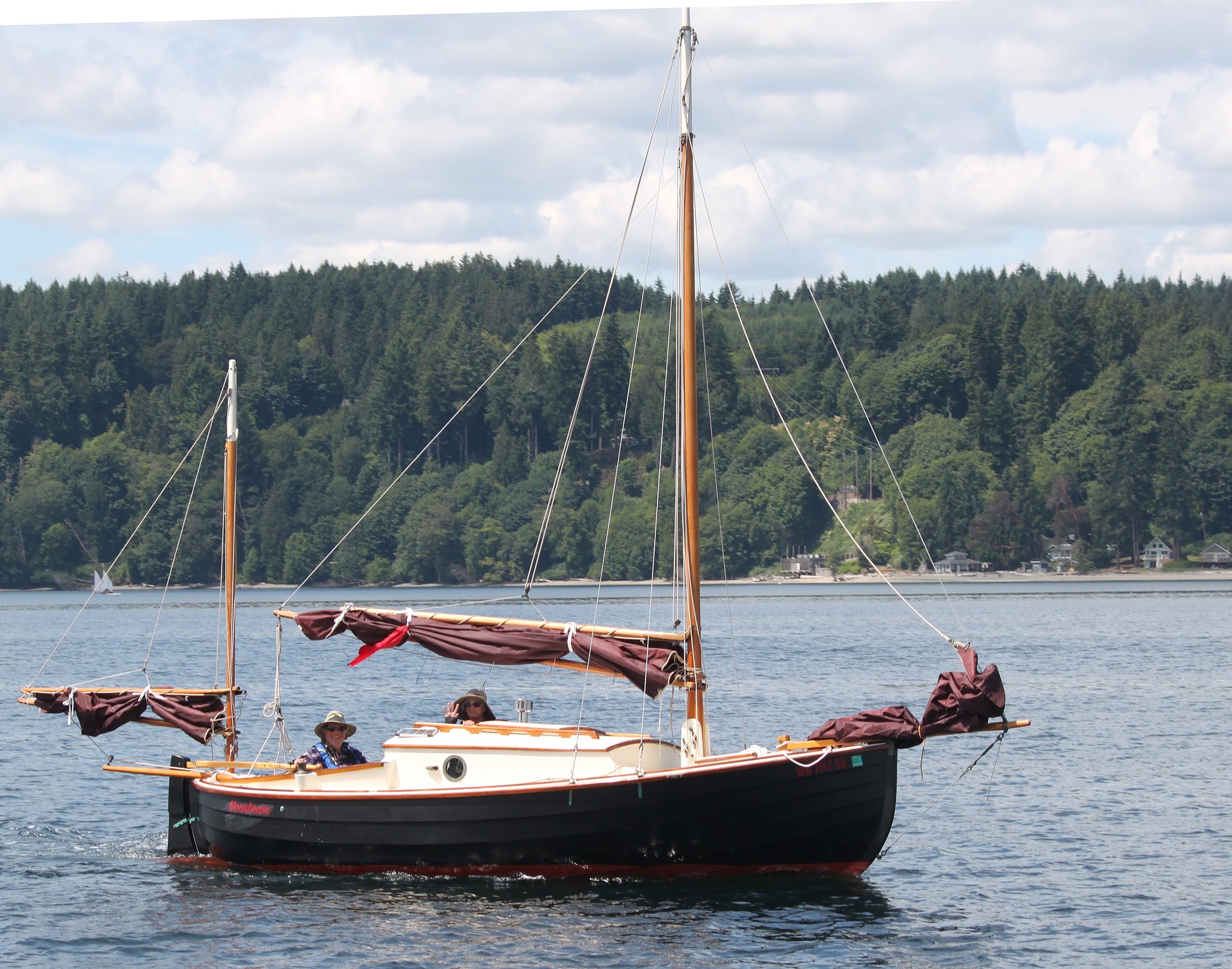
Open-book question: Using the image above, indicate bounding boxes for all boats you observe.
[142,583,156,589]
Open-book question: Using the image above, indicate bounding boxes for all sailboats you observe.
[13,10,1036,877]
[93,569,122,595]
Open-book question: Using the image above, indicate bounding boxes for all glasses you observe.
[327,726,346,732]
[464,701,481,707]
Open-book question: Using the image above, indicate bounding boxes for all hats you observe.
[314,710,357,739]
[451,689,496,720]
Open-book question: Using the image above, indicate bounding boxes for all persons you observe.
[442,689,491,726]
[286,714,371,772]
